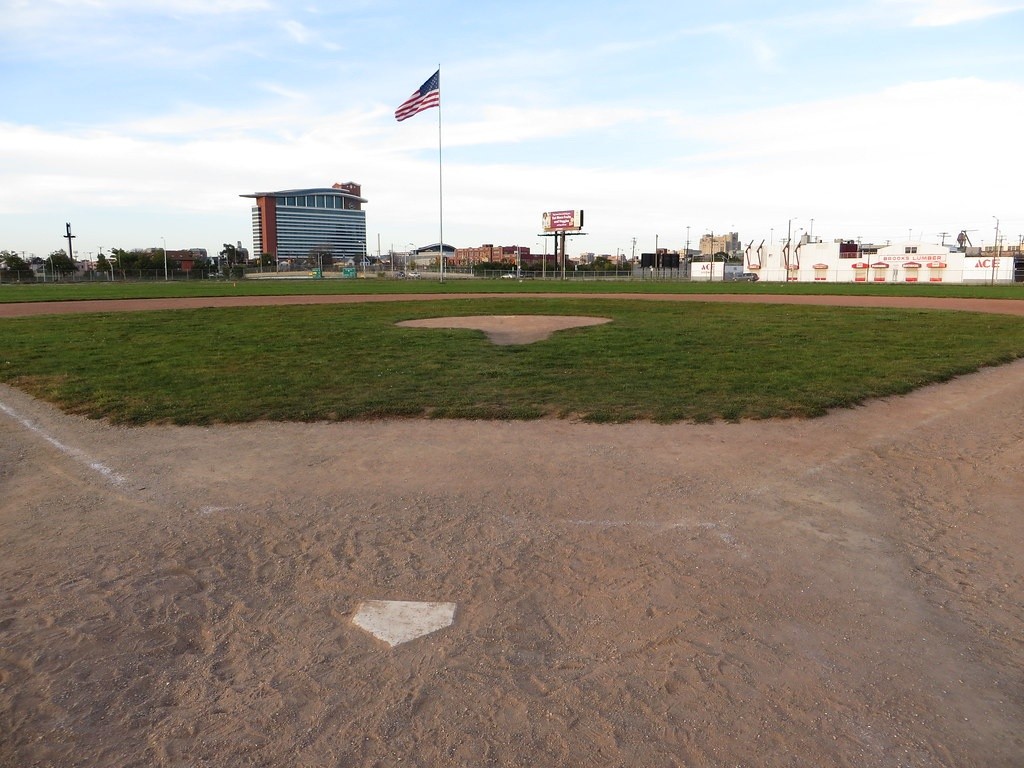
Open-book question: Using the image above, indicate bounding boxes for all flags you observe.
[395,69,440,122]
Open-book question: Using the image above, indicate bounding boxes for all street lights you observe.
[160,236,168,281]
[792,227,803,280]
[536,242,545,278]
[321,254,324,277]
[405,243,411,277]
[706,228,713,280]
[358,241,365,272]
[991,216,999,286]
[787,216,798,281]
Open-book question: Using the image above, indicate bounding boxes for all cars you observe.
[500,273,517,279]
[732,272,759,283]
[207,270,224,279]
[408,272,421,279]
[395,271,406,278]
